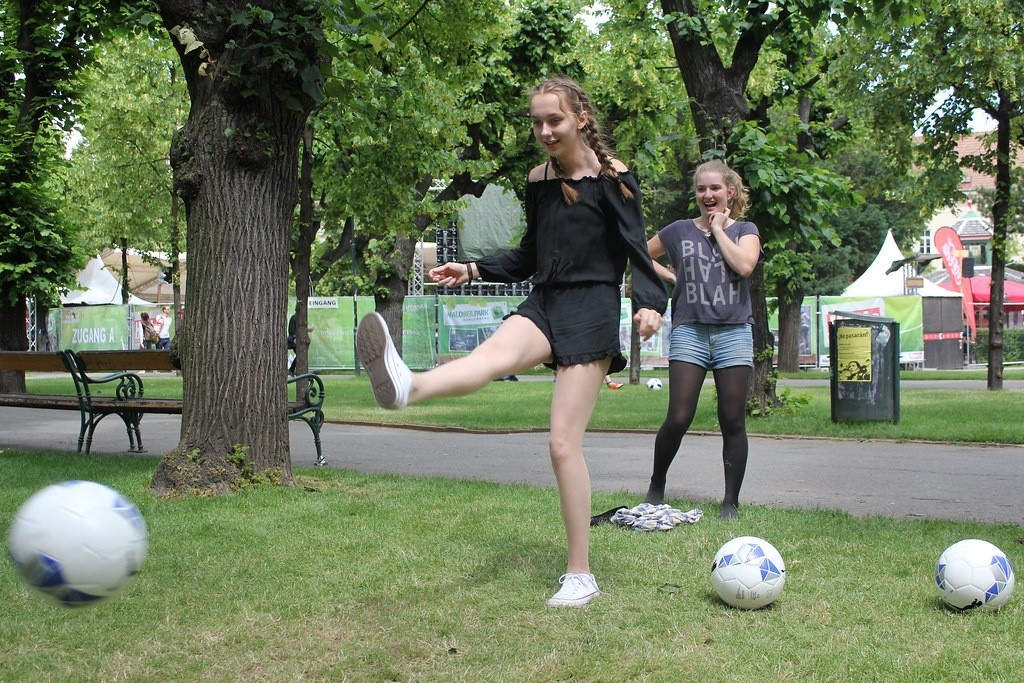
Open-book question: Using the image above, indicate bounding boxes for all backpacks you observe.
[142,322,155,340]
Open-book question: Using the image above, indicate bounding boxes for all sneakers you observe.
[355,310,411,411]
[546,573,612,607]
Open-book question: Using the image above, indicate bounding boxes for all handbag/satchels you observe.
[150,330,160,344]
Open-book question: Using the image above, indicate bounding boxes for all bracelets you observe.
[466,263,473,285]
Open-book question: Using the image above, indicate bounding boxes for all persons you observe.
[288,313,314,378]
[604,375,624,389]
[644,163,760,522]
[357,77,666,606]
[141,313,159,349]
[156,305,172,349]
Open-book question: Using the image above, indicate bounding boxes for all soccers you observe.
[935,538,1016,616]
[709,535,788,612]
[646,377,662,391]
[7,478,150,609]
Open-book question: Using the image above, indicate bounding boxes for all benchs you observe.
[0,348,329,468]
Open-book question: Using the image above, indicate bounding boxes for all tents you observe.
[940,274,1024,326]
[839,228,962,369]
[60,252,151,305]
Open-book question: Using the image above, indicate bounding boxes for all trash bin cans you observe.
[828,310,901,426]
